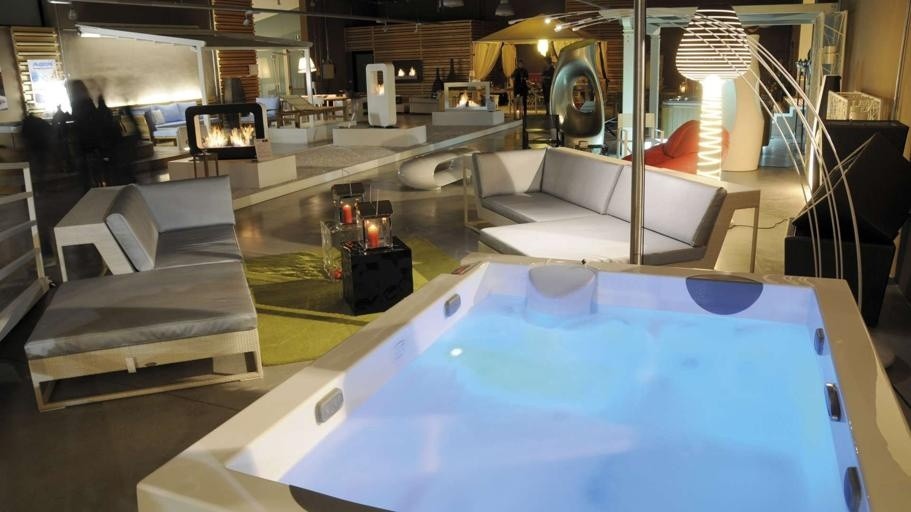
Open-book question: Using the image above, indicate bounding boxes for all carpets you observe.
[241,236,460,367]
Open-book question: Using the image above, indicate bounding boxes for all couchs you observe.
[130,100,198,146]
[464,146,761,272]
[23,174,264,414]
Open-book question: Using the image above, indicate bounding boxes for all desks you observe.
[194,153,218,179]
[319,97,350,120]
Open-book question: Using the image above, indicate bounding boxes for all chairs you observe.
[282,95,346,128]
[621,120,730,176]
[255,96,282,128]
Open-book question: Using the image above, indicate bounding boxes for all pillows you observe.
[131,101,197,125]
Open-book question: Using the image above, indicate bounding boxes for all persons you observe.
[510,60,530,117]
[64,78,130,186]
[539,57,555,116]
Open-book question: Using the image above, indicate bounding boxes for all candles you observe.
[368,225,378,248]
[343,204,352,224]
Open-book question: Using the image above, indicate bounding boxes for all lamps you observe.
[494,0,515,17]
[298,56,317,74]
